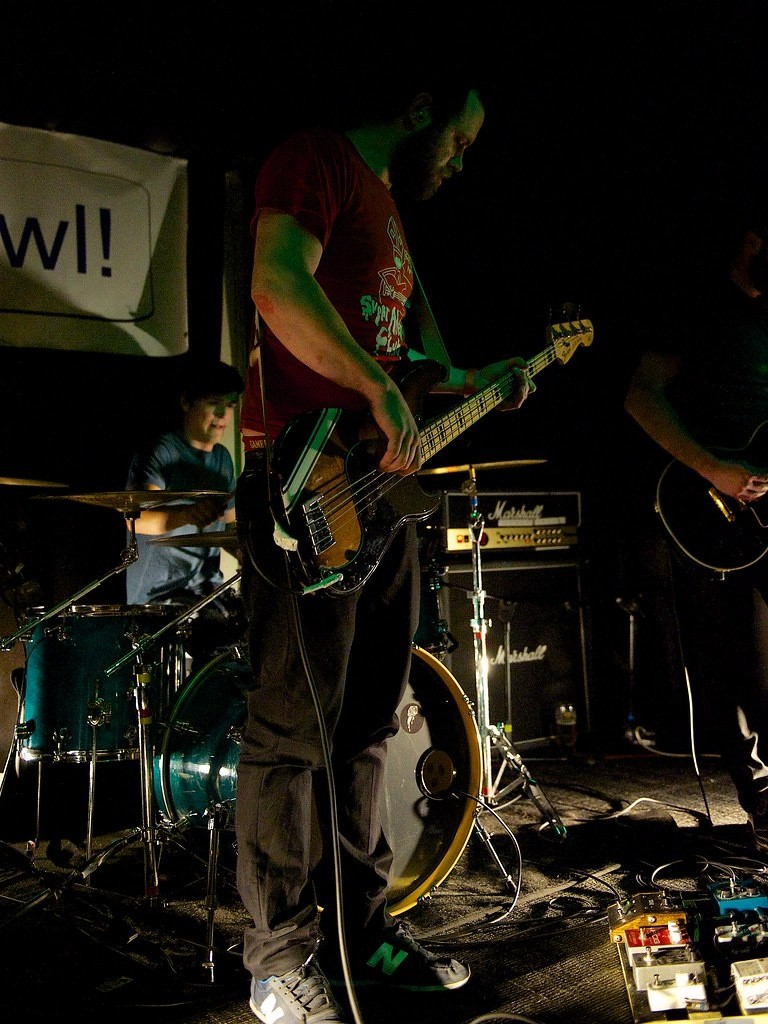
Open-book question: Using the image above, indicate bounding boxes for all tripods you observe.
[466,471,569,846]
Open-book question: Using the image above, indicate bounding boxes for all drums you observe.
[22,604,190,764]
[151,641,478,914]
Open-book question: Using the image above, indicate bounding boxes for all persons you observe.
[233,70,538,1023]
[623,201,767,854]
[123,363,240,606]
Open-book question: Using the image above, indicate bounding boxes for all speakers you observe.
[433,552,592,752]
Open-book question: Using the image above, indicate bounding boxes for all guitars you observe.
[233,317,592,599]
[658,403,768,576]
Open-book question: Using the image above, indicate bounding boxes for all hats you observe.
[185,356,245,403]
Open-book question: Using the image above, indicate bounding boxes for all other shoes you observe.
[747,807,767,841]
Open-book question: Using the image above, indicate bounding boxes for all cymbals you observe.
[48,491,227,519]
[145,530,233,550]
[0,478,71,487]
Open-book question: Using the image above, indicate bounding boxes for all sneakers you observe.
[317,921,471,992]
[249,953,355,1024]
